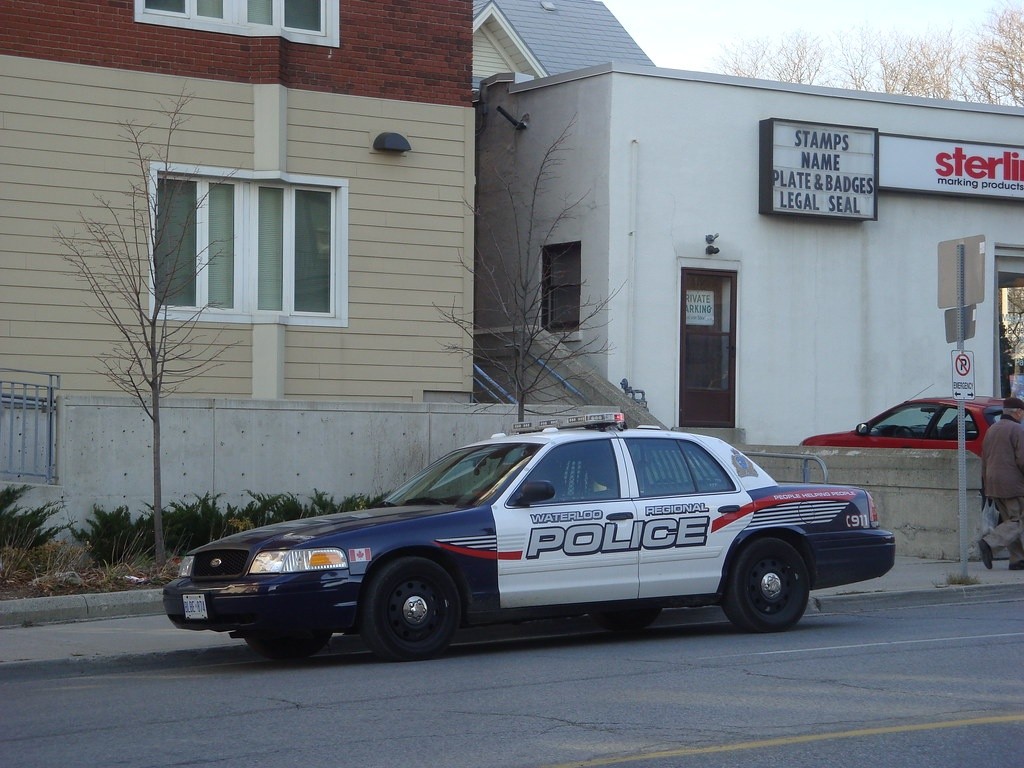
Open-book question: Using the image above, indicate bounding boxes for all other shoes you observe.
[1009,560,1024,570]
[978,539,993,569]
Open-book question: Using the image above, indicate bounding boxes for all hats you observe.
[1003,397,1024,409]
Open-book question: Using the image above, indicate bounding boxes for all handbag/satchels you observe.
[981,497,1000,536]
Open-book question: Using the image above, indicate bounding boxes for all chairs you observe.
[940,423,968,440]
[588,451,619,486]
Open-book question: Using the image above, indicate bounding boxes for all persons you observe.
[977,398,1024,570]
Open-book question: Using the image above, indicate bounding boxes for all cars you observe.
[163,411,896,663]
[797,392,1024,457]
